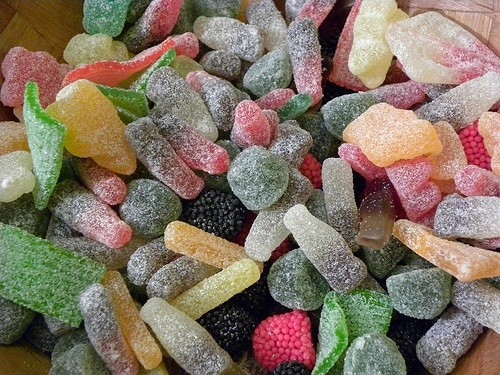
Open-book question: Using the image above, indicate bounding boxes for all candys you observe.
[1,0,500,375]
